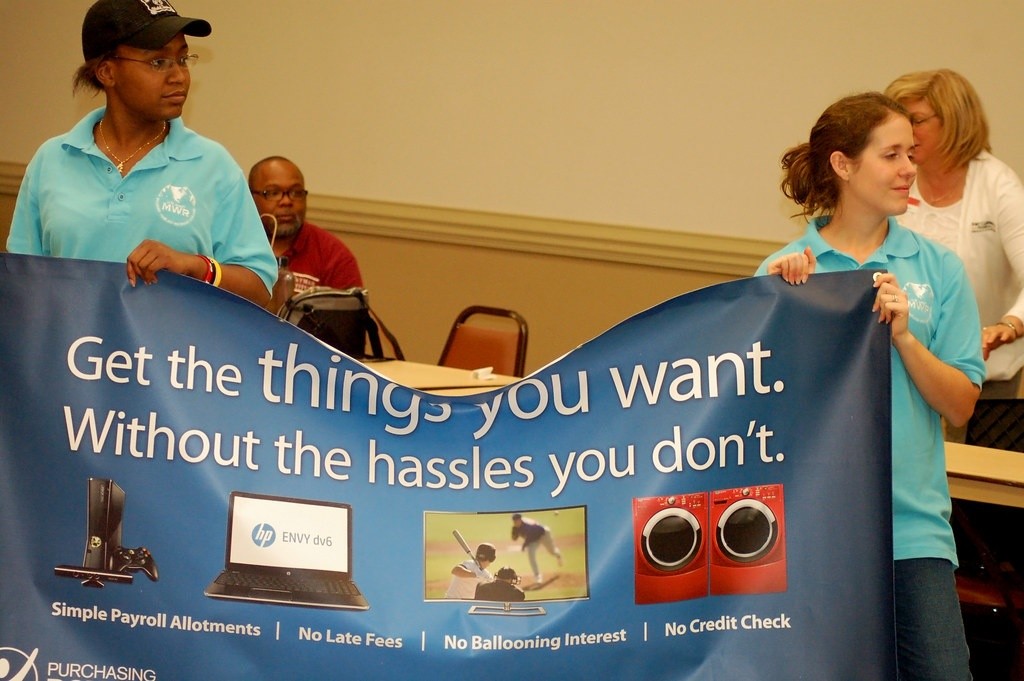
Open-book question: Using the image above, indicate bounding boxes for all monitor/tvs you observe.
[422,504,590,617]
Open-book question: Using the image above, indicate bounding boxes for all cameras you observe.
[633,483,788,605]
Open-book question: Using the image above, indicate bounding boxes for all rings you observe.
[893,294,898,302]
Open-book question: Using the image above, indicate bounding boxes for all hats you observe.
[82,0,211,62]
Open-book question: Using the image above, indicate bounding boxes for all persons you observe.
[247,156,365,293]
[753,68,1024,681]
[6,0,278,307]
[444,543,525,602]
[511,514,564,584]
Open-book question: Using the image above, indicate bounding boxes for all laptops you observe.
[203,490,371,611]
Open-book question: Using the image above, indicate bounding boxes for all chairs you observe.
[438,305,528,377]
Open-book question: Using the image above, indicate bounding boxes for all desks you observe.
[361,359,525,396]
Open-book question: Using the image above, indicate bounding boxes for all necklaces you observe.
[920,169,965,207]
[99,118,166,173]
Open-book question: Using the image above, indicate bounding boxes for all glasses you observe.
[911,113,938,128]
[251,186,308,202]
[106,54,198,72]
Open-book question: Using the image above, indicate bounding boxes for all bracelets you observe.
[996,322,1017,334]
[195,253,222,288]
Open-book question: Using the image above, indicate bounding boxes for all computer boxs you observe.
[83,478,125,572]
